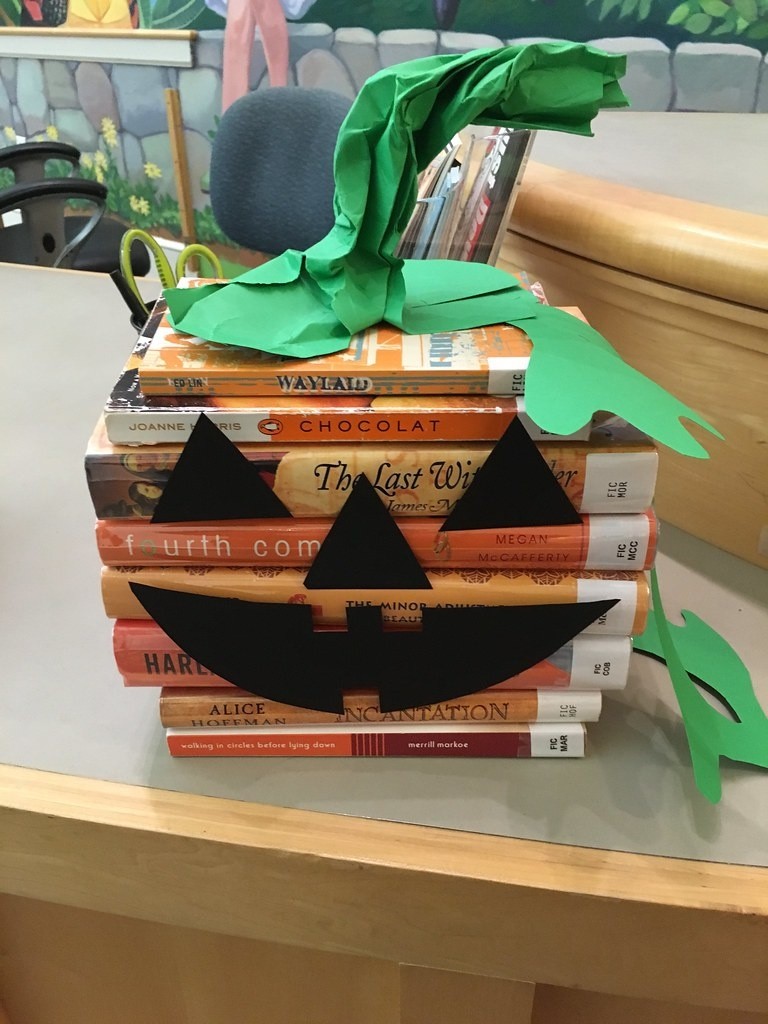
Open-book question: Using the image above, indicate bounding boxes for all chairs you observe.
[0,141,151,277]
[209,84,354,256]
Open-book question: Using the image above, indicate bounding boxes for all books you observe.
[84,276,660,758]
[395,125,539,266]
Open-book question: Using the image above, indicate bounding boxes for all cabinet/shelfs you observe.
[0,102,768,1024]
